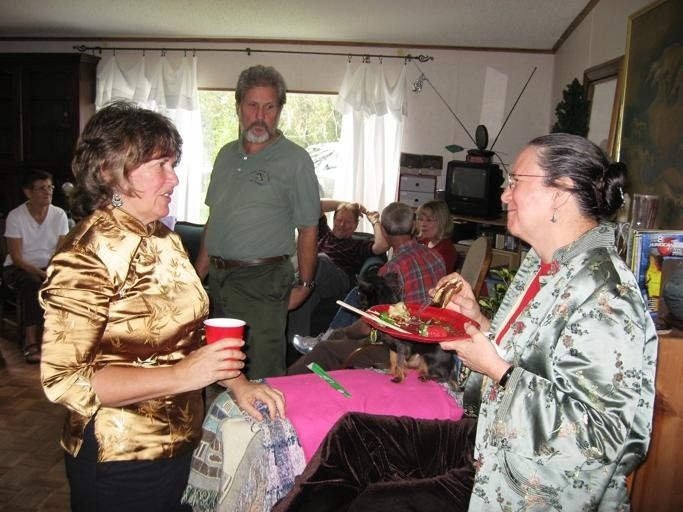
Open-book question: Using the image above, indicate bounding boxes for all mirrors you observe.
[580,54,624,162]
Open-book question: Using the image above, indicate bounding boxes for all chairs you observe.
[459,236,492,300]
[620,338,682,512]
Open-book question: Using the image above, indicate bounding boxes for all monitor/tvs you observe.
[444,159,505,218]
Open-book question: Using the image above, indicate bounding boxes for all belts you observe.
[209,256,289,267]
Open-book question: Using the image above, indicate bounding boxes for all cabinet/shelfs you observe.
[395,173,437,209]
[452,209,522,270]
[0,53,101,214]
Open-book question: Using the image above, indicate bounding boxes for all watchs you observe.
[298,280,316,289]
[497,366,516,391]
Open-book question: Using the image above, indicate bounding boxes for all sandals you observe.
[24,342,41,363]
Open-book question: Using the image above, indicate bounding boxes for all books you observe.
[630,230,683,323]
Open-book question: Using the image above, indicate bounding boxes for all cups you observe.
[203,318,246,371]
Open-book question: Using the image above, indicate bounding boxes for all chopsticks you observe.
[336,299,412,334]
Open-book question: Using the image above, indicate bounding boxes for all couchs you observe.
[173,221,384,338]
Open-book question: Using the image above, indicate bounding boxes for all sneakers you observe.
[293,333,323,355]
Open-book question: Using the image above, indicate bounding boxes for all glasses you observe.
[508,174,547,186]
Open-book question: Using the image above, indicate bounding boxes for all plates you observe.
[361,303,481,343]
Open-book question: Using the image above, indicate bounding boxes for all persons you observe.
[433,132,658,508]
[2,167,68,362]
[288,199,392,335]
[36,95,285,512]
[292,199,458,356]
[195,66,321,380]
[291,269,454,374]
[378,202,447,305]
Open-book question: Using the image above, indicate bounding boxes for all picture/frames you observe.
[606,0,683,230]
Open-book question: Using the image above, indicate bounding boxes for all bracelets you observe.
[372,219,381,224]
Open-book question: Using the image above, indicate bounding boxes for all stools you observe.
[0,269,29,344]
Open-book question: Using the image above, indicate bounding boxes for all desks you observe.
[179,367,463,512]
